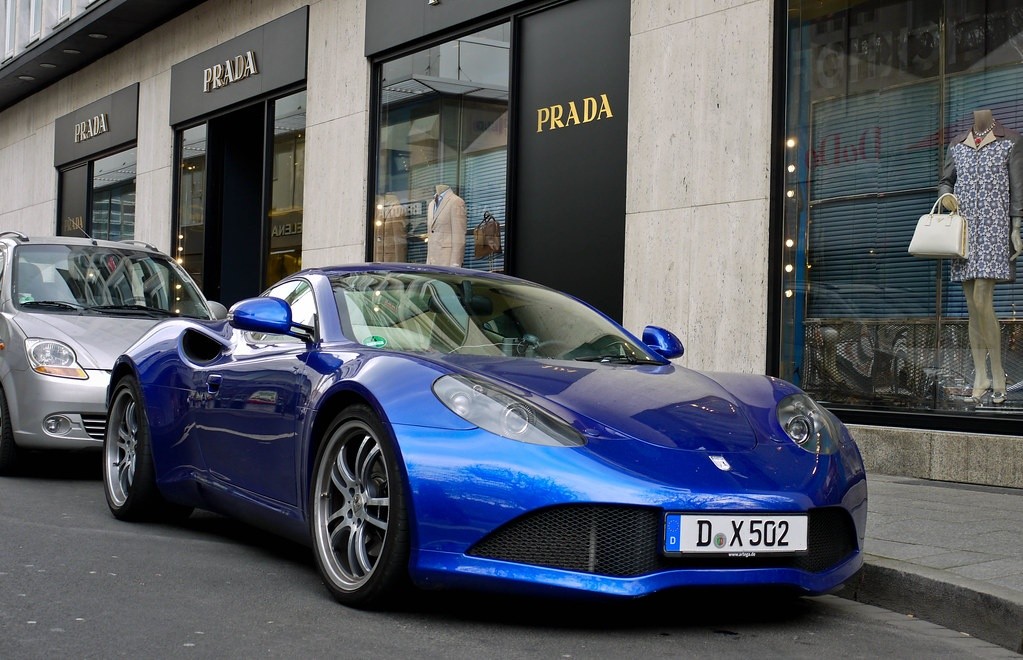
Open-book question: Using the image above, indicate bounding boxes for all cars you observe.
[0,230,227,479]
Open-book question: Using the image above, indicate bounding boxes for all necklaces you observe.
[974,119,996,136]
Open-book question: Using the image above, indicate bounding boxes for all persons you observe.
[375,194,407,262]
[938,110,1023,403]
[426,186,465,268]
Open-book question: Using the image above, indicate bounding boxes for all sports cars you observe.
[102,262,869,613]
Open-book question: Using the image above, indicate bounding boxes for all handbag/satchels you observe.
[909,195,971,260]
[474,211,502,257]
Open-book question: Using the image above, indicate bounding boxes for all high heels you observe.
[992,374,1009,404]
[965,384,992,406]
